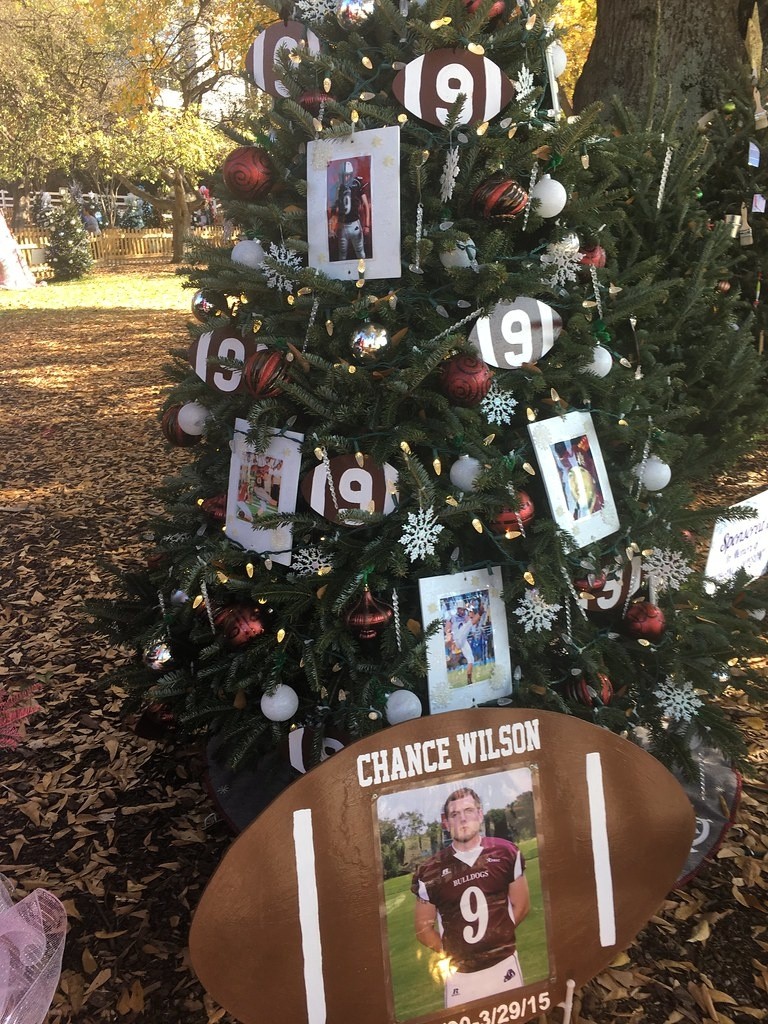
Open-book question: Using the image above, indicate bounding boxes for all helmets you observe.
[456,599,466,613]
[338,161,355,186]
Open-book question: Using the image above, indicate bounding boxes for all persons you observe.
[328,161,371,260]
[235,451,285,521]
[444,597,487,684]
[563,446,605,519]
[352,323,378,348]
[78,207,101,235]
[409,787,530,1009]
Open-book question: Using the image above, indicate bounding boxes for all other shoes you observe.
[477,602,486,616]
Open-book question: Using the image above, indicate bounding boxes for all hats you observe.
[561,446,597,480]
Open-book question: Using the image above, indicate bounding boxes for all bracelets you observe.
[365,225,370,228]
